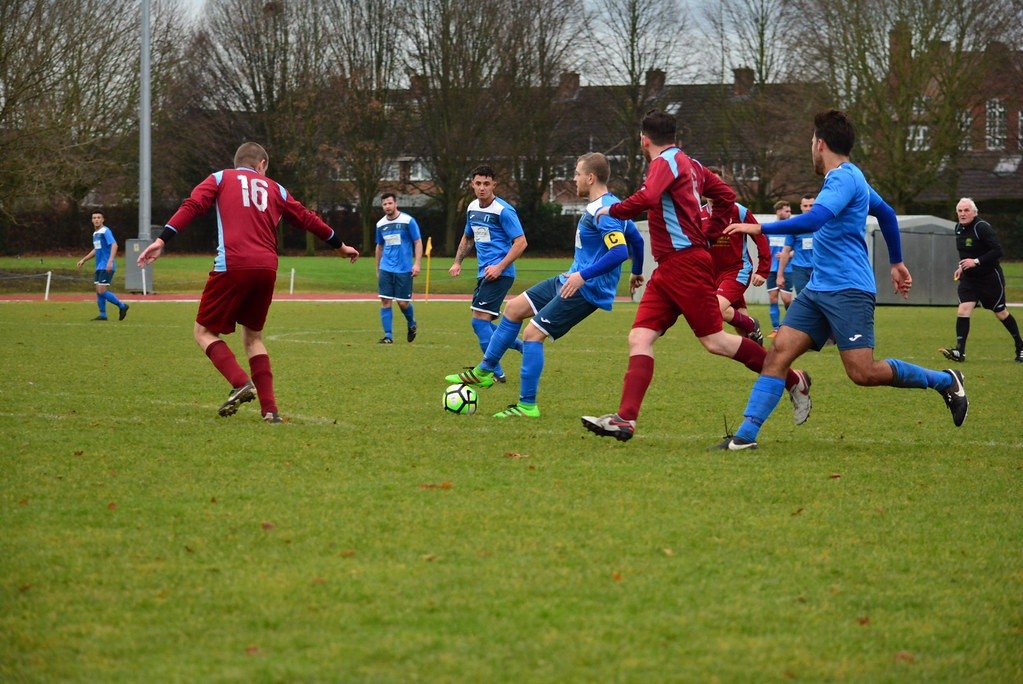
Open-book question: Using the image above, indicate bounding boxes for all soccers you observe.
[442,383,480,415]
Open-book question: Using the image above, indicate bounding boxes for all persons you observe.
[767,200,793,337]
[719,110,969,449]
[943,197,1023,362]
[445,154,644,417]
[449,165,528,389]
[776,193,816,294]
[700,166,771,349]
[374,193,423,344]
[582,107,813,442]
[136,142,360,425]
[76,209,129,321]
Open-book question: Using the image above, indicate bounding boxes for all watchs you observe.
[974,259,979,266]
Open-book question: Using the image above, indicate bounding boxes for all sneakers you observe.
[379,337,393,344]
[943,348,965,362]
[1015,342,1023,361]
[745,317,763,346]
[263,412,283,426]
[444,367,494,389]
[766,330,778,338]
[581,413,636,442]
[492,373,506,383]
[91,316,107,320]
[218,381,257,417]
[491,404,540,418]
[705,414,758,450]
[789,370,812,425]
[407,322,416,342]
[939,369,969,428]
[119,304,130,320]
